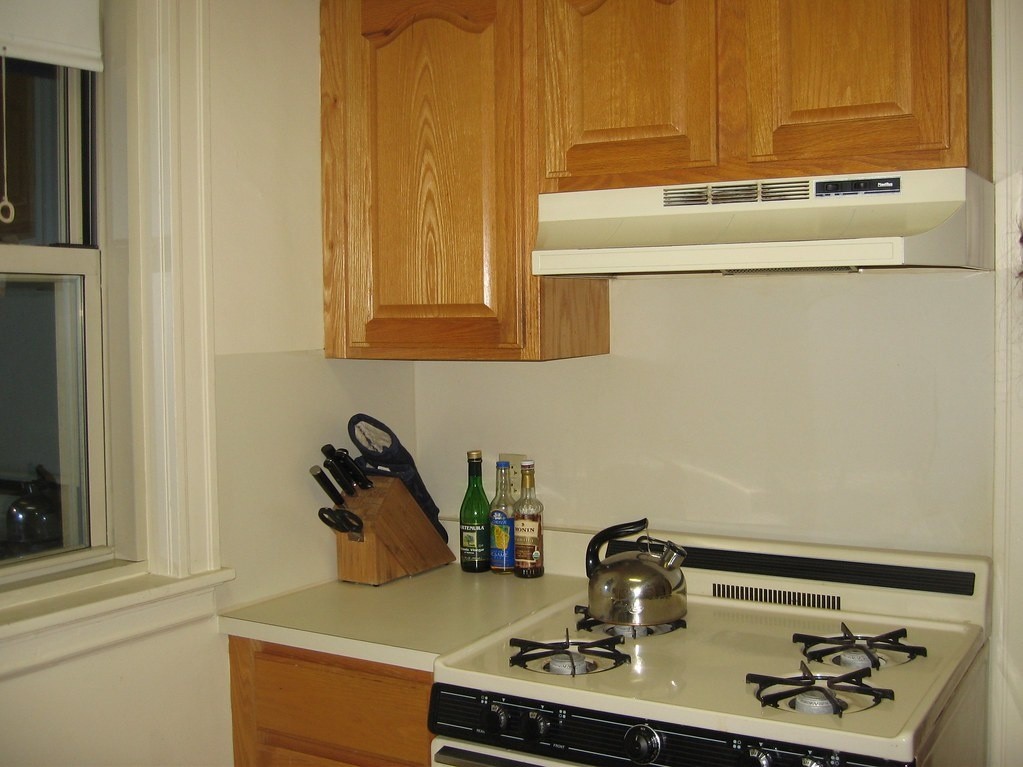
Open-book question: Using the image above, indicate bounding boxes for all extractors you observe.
[531,168,995,279]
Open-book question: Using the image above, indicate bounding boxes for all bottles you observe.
[459,450,490,573]
[489,461,515,575]
[514,460,544,578]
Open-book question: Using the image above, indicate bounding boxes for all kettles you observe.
[5,480,62,543]
[586,518,688,626]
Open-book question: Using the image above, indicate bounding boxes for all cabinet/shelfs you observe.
[317,1,990,363]
[228,633,435,767]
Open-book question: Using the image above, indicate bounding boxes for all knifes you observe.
[310,444,375,511]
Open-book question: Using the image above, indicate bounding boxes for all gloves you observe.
[347,413,448,543]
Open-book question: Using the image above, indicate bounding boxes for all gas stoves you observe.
[428,529,983,767]
